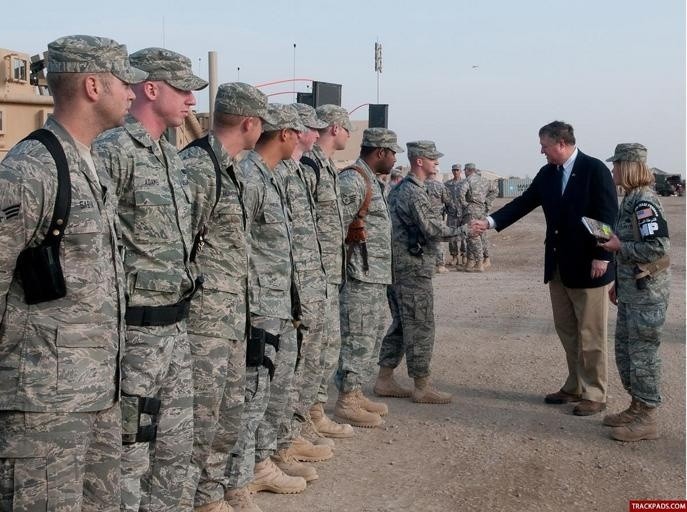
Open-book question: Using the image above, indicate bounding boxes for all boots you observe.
[194,402,354,512]
[437,255,492,273]
[412,376,453,404]
[334,391,390,426]
[373,366,413,398]
[602,399,660,441]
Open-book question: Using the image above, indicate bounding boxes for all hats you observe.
[129,47,208,91]
[391,168,405,179]
[214,82,354,133]
[605,143,648,162]
[407,140,445,161]
[46,35,150,85]
[452,163,482,177]
[361,128,405,154]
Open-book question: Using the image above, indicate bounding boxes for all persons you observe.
[301,102,357,446]
[375,162,500,275]
[663,179,685,198]
[338,126,405,429]
[600,142,671,442]
[240,104,319,506]
[267,103,336,482]
[92,47,211,512]
[467,119,618,416]
[0,35,151,512]
[374,141,485,405]
[177,82,277,511]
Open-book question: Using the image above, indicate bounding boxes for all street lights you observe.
[190,37,298,103]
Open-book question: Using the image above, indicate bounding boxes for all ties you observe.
[556,164,565,200]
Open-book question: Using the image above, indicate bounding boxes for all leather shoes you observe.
[544,388,582,403]
[573,399,607,416]
